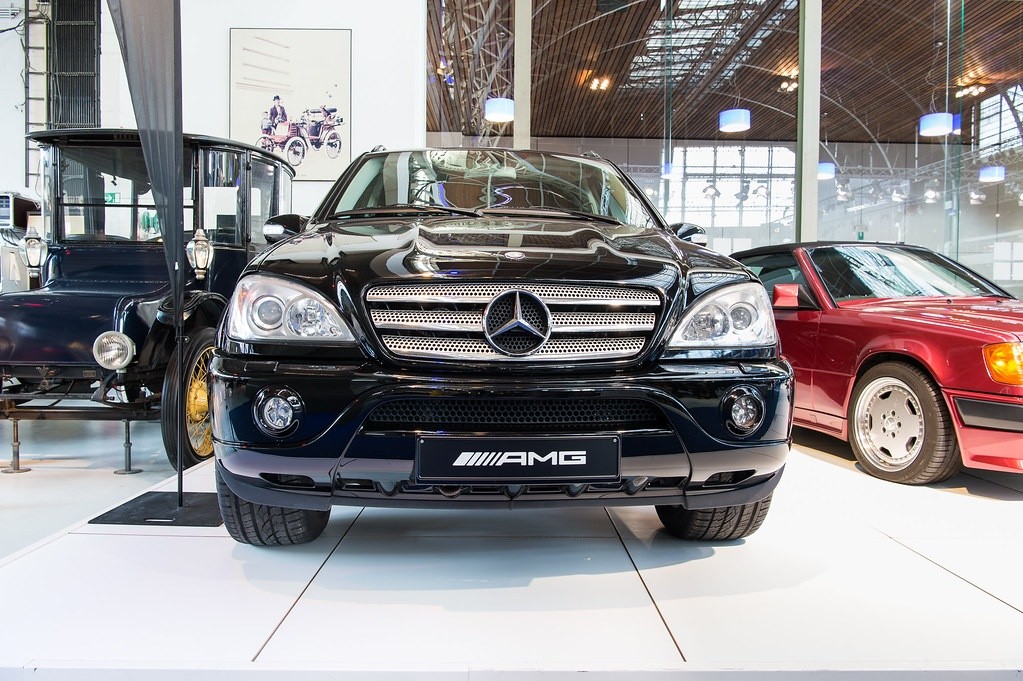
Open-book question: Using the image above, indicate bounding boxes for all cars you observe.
[0,129,325,471]
[249,104,344,165]
[729,240,1023,487]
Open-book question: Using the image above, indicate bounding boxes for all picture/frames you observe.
[227,27,352,183]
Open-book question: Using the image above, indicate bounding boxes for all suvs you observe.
[205,145,801,543]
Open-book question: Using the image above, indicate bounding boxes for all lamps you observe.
[918,41,953,137]
[776,77,797,93]
[955,83,986,98]
[590,77,608,90]
[642,148,1005,216]
[719,36,750,133]
[485,31,514,123]
